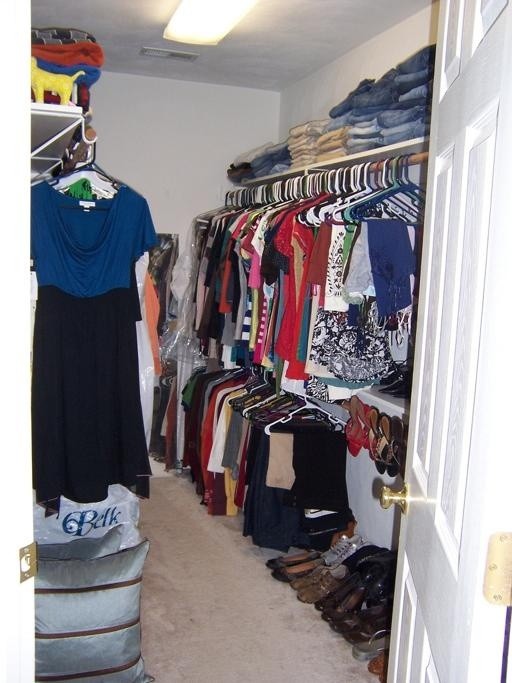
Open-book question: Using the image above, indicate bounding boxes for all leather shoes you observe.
[267,535,388,681]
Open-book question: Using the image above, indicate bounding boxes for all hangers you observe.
[192,151,426,232]
[190,352,351,439]
[31,121,127,215]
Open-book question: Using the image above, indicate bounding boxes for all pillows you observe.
[33,523,156,683]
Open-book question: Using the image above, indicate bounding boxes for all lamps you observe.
[163,0,257,45]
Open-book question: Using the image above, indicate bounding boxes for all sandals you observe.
[343,371,408,476]
